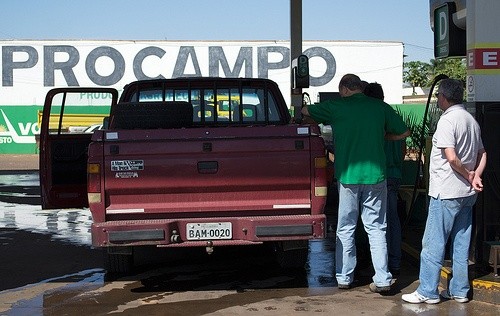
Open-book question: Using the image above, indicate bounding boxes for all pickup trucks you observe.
[38,76,330,279]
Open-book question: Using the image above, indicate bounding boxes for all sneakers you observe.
[368,282,392,294]
[337,281,352,289]
[401,290,441,304]
[441,289,469,302]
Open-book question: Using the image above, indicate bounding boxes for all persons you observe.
[300,74,411,294]
[400,79,487,305]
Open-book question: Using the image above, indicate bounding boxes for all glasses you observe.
[434,90,444,98]
